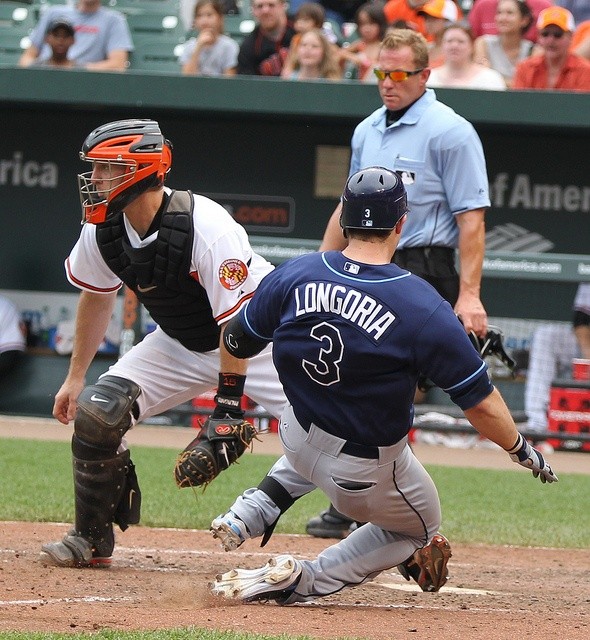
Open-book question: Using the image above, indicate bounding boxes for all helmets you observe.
[76,118,173,225]
[339,167,409,239]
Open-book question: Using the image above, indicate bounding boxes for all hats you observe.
[569,21,590,59]
[47,18,74,35]
[536,5,575,32]
[416,0,463,23]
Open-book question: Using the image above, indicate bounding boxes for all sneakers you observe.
[40,527,112,568]
[209,510,251,552]
[305,512,362,539]
[207,553,302,603]
[397,532,452,593]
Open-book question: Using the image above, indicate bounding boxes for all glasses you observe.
[542,30,563,39]
[373,67,423,81]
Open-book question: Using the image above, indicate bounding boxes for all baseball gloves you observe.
[174,415,264,488]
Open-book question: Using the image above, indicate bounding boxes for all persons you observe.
[573,284,590,360]
[29,18,87,71]
[208,166,559,605]
[468,0,555,43]
[474,0,547,90]
[237,1,385,81]
[426,25,507,91]
[383,0,460,68]
[173,0,239,75]
[305,29,491,539]
[19,0,134,70]
[39,119,452,592]
[511,6,590,91]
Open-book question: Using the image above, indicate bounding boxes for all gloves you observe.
[211,395,245,471]
[505,432,559,484]
